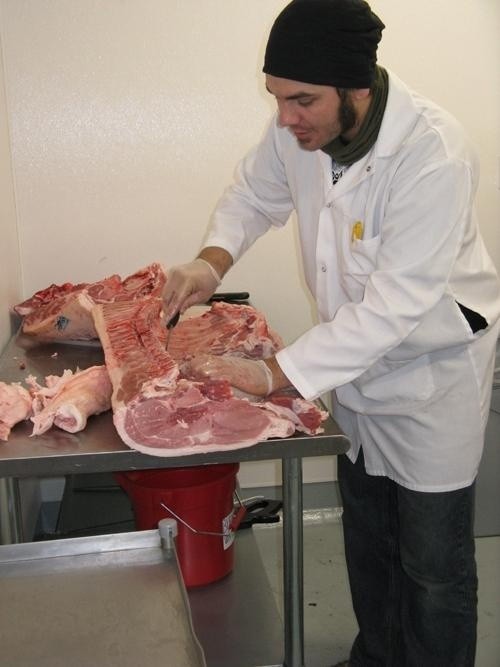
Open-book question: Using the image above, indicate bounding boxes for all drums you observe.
[165,311,180,353]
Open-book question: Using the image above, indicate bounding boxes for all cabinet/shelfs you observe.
[2,293,348,664]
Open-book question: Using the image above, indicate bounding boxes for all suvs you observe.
[115,464,245,586]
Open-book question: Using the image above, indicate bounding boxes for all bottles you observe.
[262,0,388,92]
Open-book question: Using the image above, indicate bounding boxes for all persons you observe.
[153,1,500,666]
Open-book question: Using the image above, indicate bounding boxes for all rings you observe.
[351,222,362,244]
